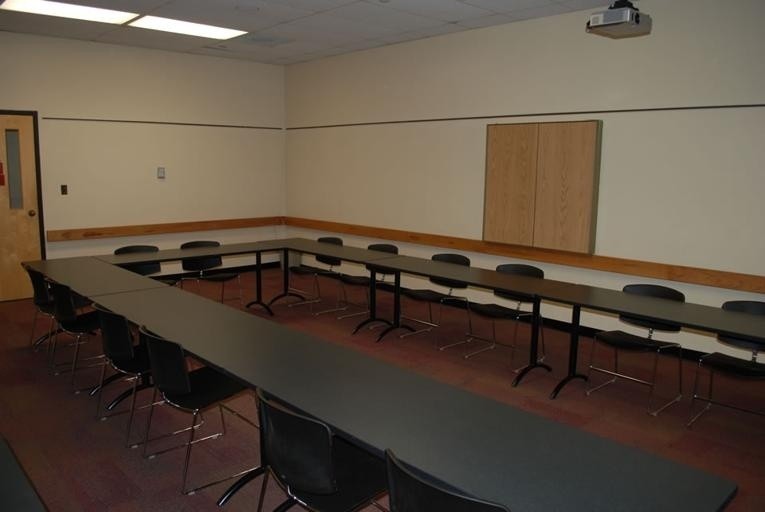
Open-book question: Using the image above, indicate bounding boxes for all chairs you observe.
[335,244,405,332]
[463,264,547,373]
[47,280,108,396]
[685,301,764,428]
[385,448,510,512]
[398,254,472,352]
[252,385,388,512]
[584,283,686,416]
[179,240,245,307]
[91,302,204,450]
[286,236,348,316]
[139,328,260,495]
[27,264,105,366]
[114,245,176,284]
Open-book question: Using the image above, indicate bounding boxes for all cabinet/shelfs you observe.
[480,119,603,256]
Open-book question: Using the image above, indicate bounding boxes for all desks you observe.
[91,242,285,317]
[257,237,406,334]
[536,285,764,400]
[87,285,741,512]
[21,256,171,351]
[364,256,580,387]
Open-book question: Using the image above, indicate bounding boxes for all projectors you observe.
[590,7,652,39]
